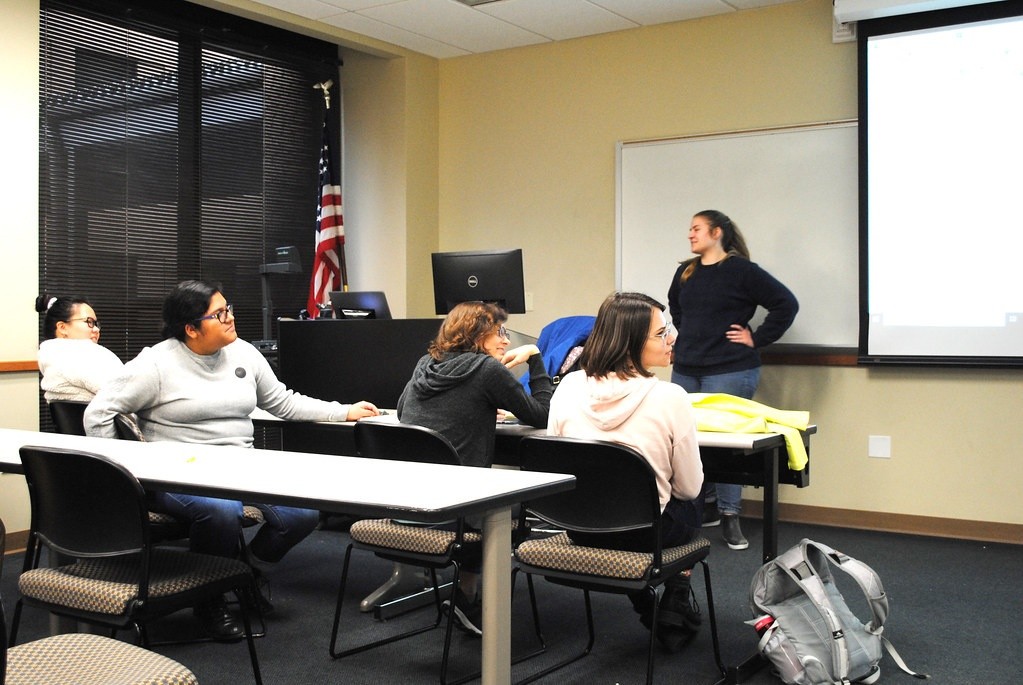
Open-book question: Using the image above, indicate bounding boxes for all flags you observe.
[306,109,348,319]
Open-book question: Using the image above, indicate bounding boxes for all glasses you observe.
[645,319,671,341]
[183,304,233,327]
[493,326,510,340]
[55,317,101,330]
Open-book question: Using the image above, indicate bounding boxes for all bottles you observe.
[743,612,777,640]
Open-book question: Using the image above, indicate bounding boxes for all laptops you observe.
[329,291,393,320]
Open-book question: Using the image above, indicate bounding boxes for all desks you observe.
[248,404,817,685]
[1,429,578,685]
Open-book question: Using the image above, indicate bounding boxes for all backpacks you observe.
[748,538,888,685]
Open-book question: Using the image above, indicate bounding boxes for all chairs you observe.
[326,421,547,685]
[510,435,729,684]
[0,399,264,685]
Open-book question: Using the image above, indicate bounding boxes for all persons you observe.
[668,210,799,549]
[546,291,705,651]
[36,293,142,442]
[398,301,553,636]
[83,281,378,639]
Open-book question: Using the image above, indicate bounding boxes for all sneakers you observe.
[442,588,483,638]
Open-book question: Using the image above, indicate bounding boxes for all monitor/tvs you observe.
[432,249,525,315]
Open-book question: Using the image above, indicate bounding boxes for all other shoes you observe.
[693,499,721,528]
[722,514,749,550]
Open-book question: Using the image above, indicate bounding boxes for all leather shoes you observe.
[193,593,244,641]
[232,572,281,619]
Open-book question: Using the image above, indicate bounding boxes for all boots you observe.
[653,574,702,633]
[641,609,697,652]
